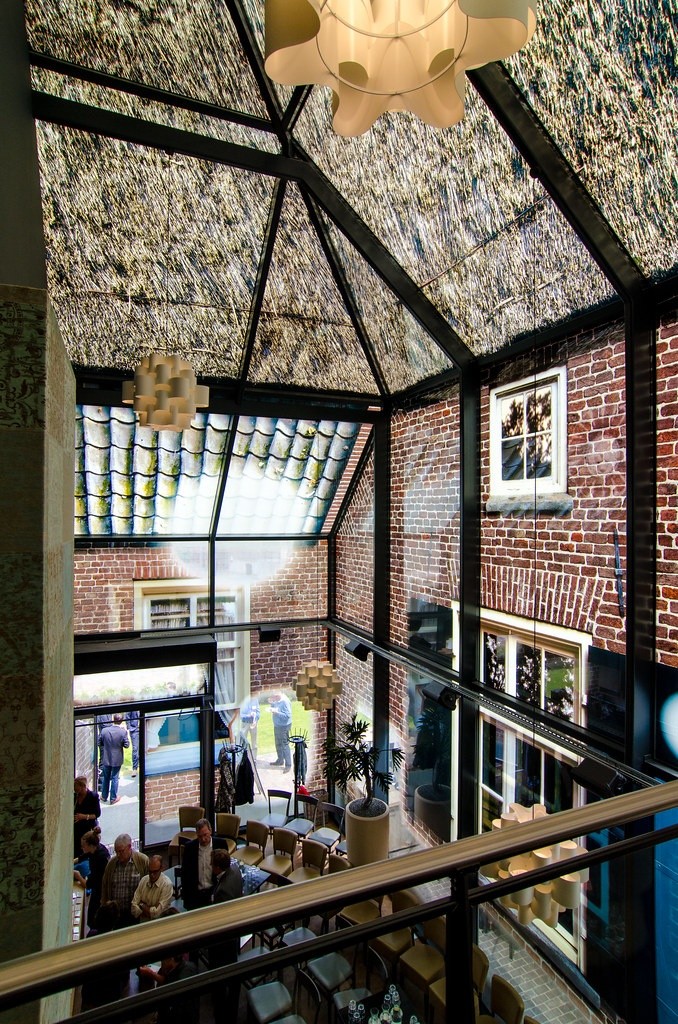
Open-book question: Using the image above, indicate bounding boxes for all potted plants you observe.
[411,701,452,834]
[320,712,412,866]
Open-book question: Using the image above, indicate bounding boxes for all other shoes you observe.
[110,800,116,804]
[100,797,107,802]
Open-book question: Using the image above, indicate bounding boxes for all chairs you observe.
[166,786,542,1024]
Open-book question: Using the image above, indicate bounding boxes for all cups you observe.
[230,856,261,885]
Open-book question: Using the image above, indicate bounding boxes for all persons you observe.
[181,819,229,910]
[241,698,260,766]
[100,834,150,905]
[81,901,130,1010]
[209,849,244,904]
[266,690,292,774]
[73,827,111,928]
[74,711,140,792]
[136,907,215,985]
[98,714,130,804]
[74,777,101,880]
[131,855,173,922]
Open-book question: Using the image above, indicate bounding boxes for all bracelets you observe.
[87,814,89,820]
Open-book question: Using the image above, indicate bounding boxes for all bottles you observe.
[368,984,403,1024]
[410,1016,421,1024]
[348,1000,366,1024]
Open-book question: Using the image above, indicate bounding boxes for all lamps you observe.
[120,143,212,433]
[423,681,459,713]
[262,0,540,138]
[342,640,371,663]
[258,624,282,643]
[572,754,625,801]
[481,789,591,931]
[290,418,344,712]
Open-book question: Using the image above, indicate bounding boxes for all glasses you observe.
[114,847,128,854]
[148,866,161,875]
